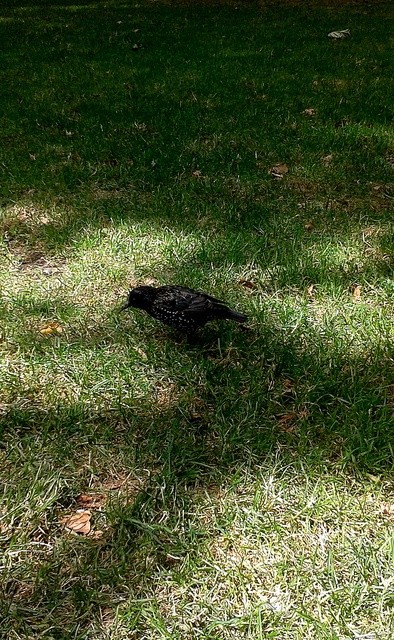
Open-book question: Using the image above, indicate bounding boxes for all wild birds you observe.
[118,285,248,346]
